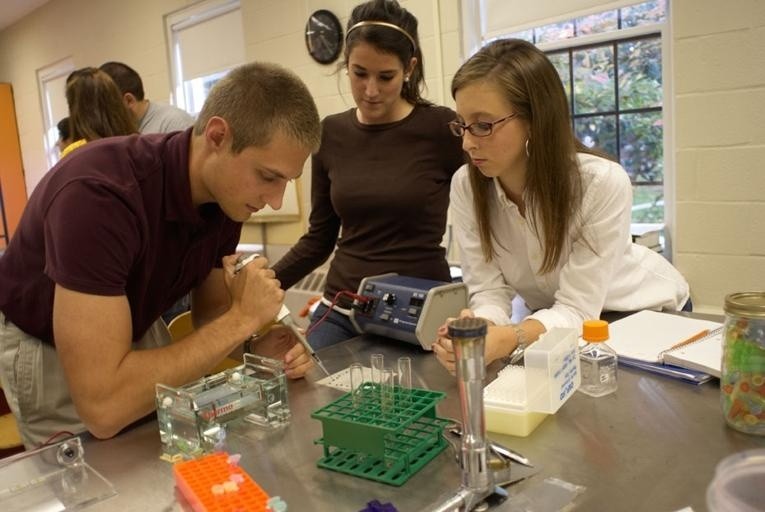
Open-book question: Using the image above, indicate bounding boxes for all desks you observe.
[1,312,765,512]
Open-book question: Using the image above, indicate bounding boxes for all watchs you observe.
[500,323,529,364]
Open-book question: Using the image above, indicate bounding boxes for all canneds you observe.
[721,292,765,437]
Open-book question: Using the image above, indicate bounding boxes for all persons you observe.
[270,0,470,351]
[0,60,317,453]
[54,60,197,157]
[431,38,692,377]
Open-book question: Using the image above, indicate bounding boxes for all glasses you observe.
[448,113,518,138]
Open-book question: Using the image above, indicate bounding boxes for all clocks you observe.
[303,8,344,66]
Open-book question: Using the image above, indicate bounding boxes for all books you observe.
[580,309,725,387]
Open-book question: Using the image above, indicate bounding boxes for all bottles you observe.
[379,367,395,473]
[349,362,366,409]
[396,356,412,443]
[577,319,619,398]
[371,353,384,396]
